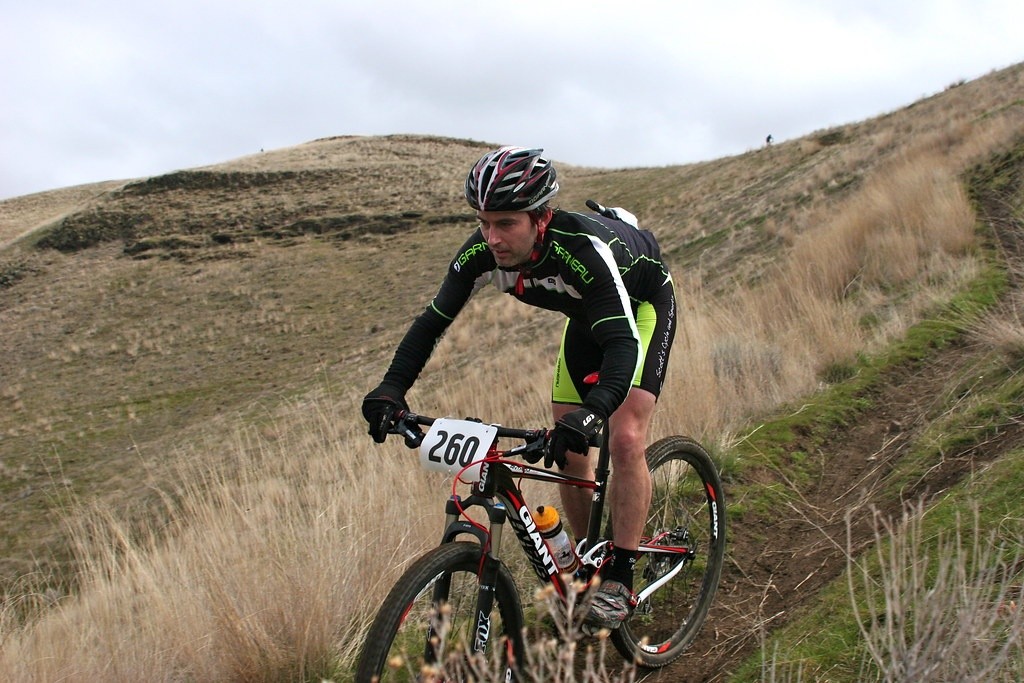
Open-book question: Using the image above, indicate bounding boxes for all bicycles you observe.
[354,409,727,683]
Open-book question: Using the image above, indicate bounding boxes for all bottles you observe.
[532,505,578,575]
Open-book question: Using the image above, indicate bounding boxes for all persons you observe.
[361,145,677,630]
[767,135,771,145]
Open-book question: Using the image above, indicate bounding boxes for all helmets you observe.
[464,146,559,211]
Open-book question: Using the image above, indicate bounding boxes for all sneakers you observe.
[574,579,641,630]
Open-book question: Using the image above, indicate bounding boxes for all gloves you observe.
[544,410,603,470]
[362,384,422,444]
